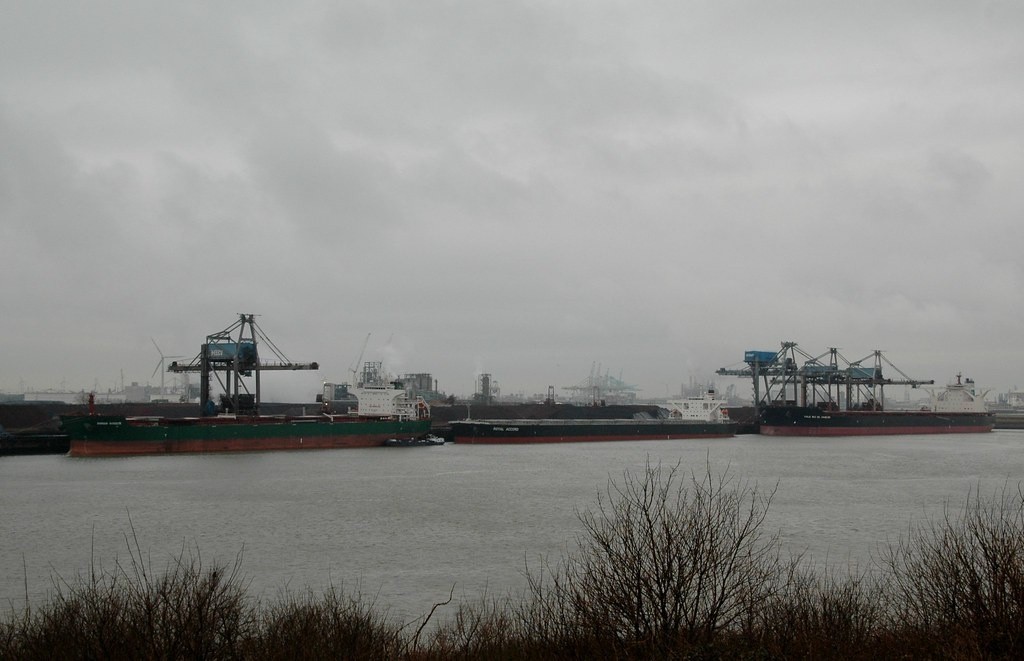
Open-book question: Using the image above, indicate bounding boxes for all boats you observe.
[446,387,743,444]
[758,371,995,439]
[56,384,432,458]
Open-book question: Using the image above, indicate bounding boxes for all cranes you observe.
[165,314,319,418]
[341,330,371,385]
[715,339,936,419]
